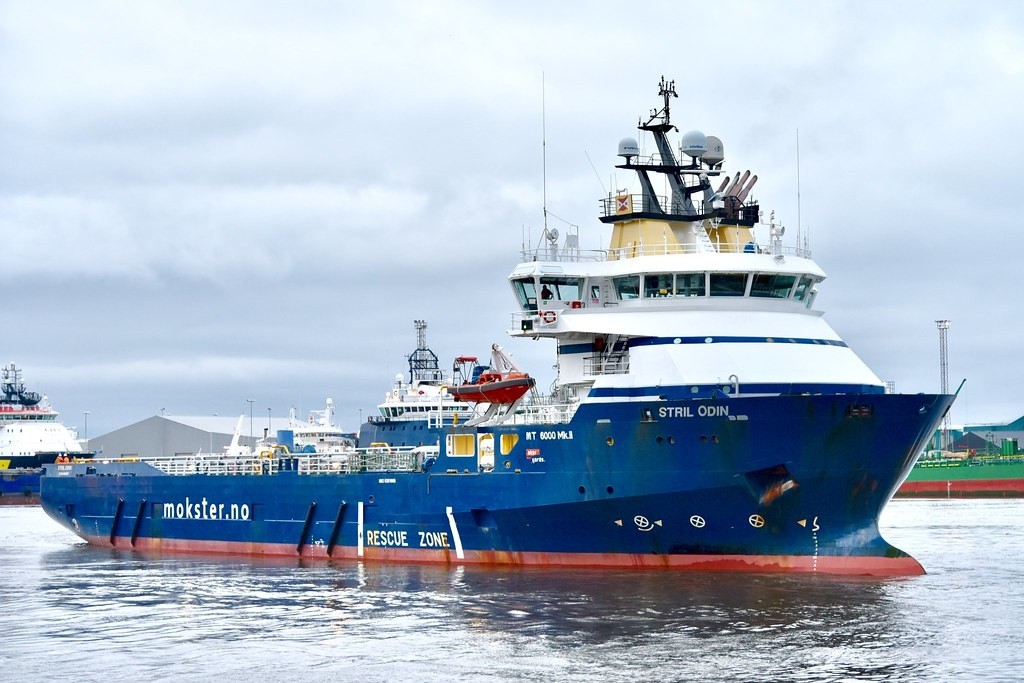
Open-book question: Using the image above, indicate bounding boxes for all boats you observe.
[446,344,537,404]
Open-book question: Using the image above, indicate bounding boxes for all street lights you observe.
[83,411,90,441]
[246,400,257,439]
[358,408,362,426]
[267,408,273,435]
[210,411,218,454]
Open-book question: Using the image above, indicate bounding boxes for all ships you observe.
[38,69,966,580]
[0,360,103,505]
